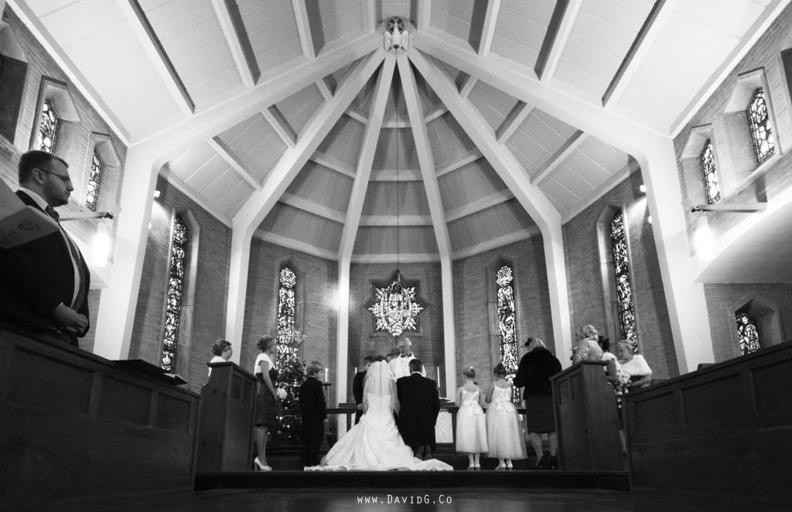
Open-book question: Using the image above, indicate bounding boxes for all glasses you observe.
[39,168,69,184]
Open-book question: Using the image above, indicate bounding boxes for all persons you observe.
[485,364,528,470]
[352,355,374,425]
[599,335,628,453]
[616,339,651,393]
[0,151,92,348]
[390,337,427,380]
[254,335,281,468]
[207,340,232,380]
[393,359,440,461]
[455,366,490,471]
[304,357,454,470]
[572,325,603,366]
[298,361,329,469]
[512,338,563,471]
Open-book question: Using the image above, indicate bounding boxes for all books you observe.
[0,179,61,249]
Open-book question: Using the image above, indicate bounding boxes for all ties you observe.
[46,206,88,312]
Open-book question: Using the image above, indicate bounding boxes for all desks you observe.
[622,338,791,511]
[326,400,458,452]
[0,329,198,507]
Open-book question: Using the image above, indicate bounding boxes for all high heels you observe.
[532,456,547,470]
[507,463,514,470]
[467,465,474,472]
[494,464,505,472]
[549,458,557,470]
[474,465,480,471]
[254,457,272,472]
